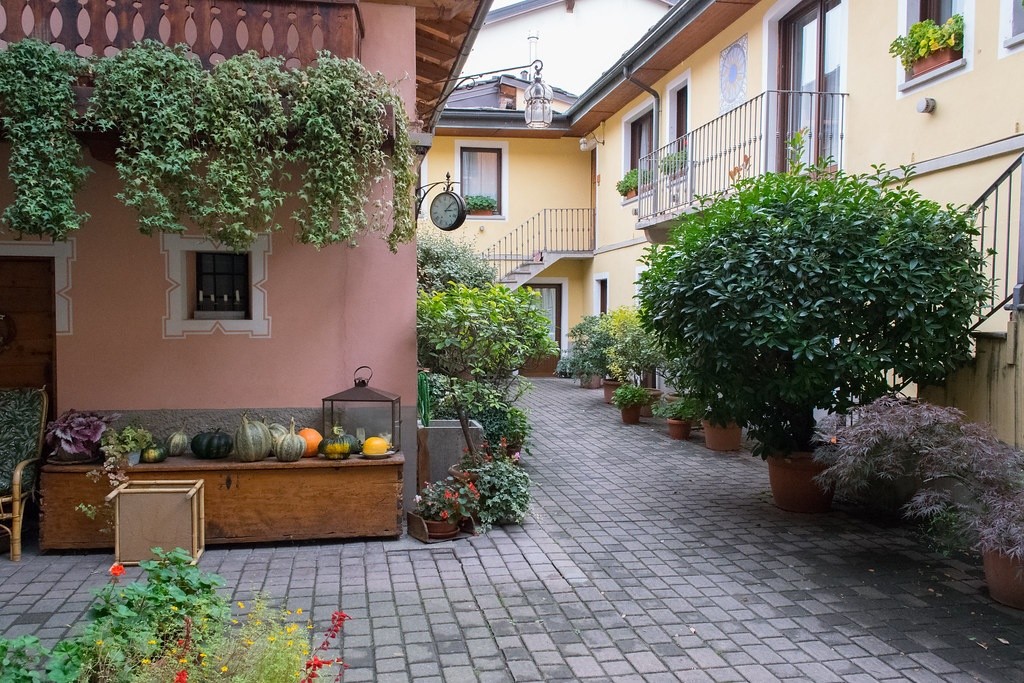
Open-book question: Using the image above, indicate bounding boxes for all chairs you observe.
[0,387,49,561]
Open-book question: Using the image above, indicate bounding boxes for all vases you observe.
[425,520,461,539]
[981,542,1024,610]
[447,464,479,484]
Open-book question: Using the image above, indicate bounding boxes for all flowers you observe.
[412,436,532,535]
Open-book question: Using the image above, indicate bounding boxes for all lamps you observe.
[416,60,553,134]
[579,130,605,151]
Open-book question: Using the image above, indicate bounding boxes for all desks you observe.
[38,452,406,550]
[104,480,204,571]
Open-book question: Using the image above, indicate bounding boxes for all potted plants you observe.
[0,35,415,255]
[553,308,743,452]
[461,195,498,216]
[236,290,240,301]
[888,14,965,78]
[616,167,652,200]
[657,151,689,181]
[45,408,121,462]
[632,127,1001,515]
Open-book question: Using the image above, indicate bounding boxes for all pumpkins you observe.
[140,409,388,462]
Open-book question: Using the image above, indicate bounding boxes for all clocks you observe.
[429,172,467,231]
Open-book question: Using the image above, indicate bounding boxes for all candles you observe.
[211,294,214,301]
[223,294,227,301]
[198,290,203,301]
